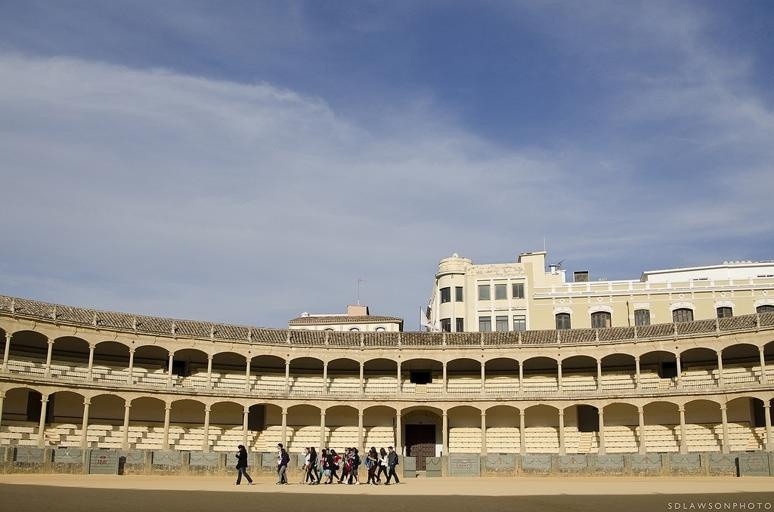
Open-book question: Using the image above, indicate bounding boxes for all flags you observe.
[421,309,441,331]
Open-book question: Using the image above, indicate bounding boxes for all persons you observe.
[275,443,290,486]
[233,445,254,485]
[302,446,400,486]
